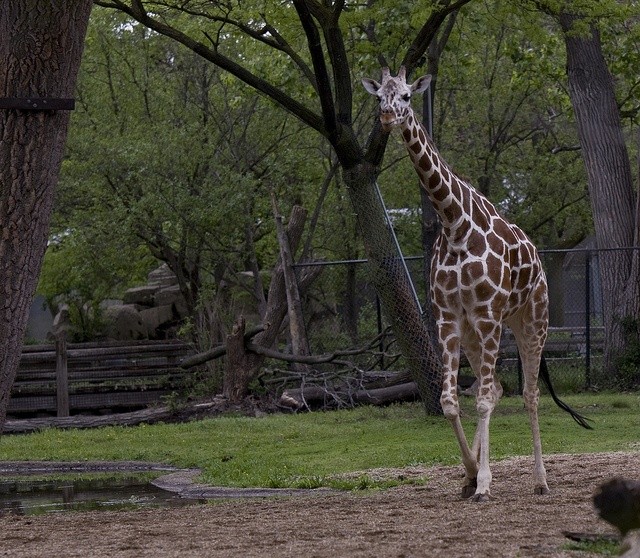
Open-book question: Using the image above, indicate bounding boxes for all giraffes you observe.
[361,64,596,504]
